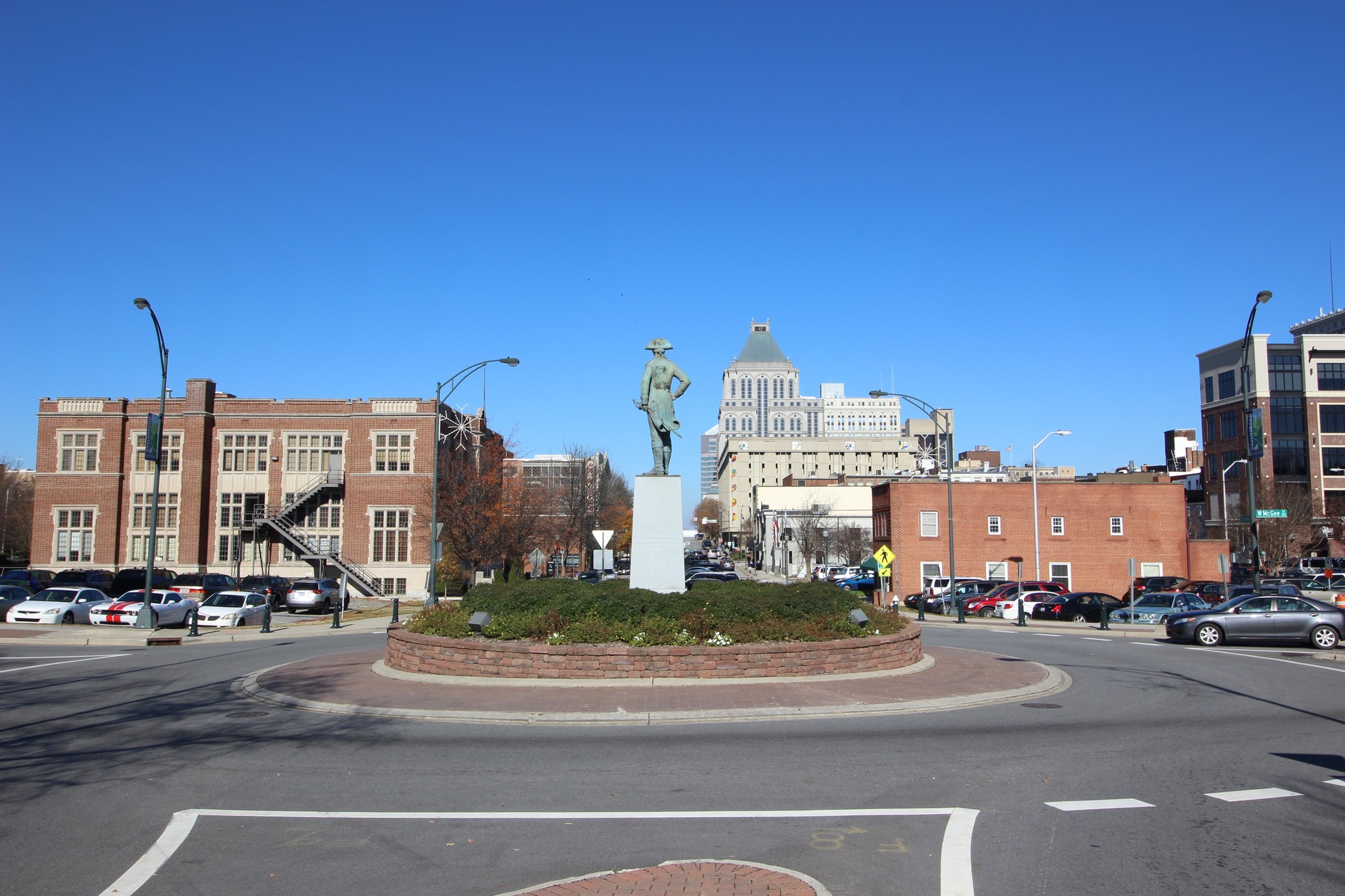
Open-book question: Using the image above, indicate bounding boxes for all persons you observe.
[690,534,738,558]
[640,338,692,476]
[747,555,751,565]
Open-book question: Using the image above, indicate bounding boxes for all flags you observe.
[145,413,161,462]
[770,510,787,544]
[1005,446,1011,451]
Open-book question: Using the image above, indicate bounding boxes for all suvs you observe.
[684,548,741,590]
[108,566,178,599]
[698,535,703,540]
[703,543,713,550]
[286,576,351,616]
[812,565,876,592]
[577,560,630,583]
[1,568,57,600]
[702,541,711,546]
[49,567,119,597]
[167,572,242,605]
[236,573,293,612]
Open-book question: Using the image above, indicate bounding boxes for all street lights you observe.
[1029,428,1072,582]
[420,355,521,612]
[753,538,757,576]
[1221,459,1248,541]
[700,508,723,551]
[713,506,731,556]
[129,293,170,631]
[784,530,790,584]
[822,526,829,582]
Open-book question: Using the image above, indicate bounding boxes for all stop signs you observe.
[719,538,723,543]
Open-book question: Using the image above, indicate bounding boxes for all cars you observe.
[1,584,45,625]
[4,586,118,625]
[186,590,272,629]
[907,555,1345,651]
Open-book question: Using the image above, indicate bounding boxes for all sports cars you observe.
[90,588,199,631]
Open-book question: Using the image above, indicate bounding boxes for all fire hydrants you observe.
[890,594,900,616]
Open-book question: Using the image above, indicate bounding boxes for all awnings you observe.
[753,543,763,552]
[746,537,757,548]
